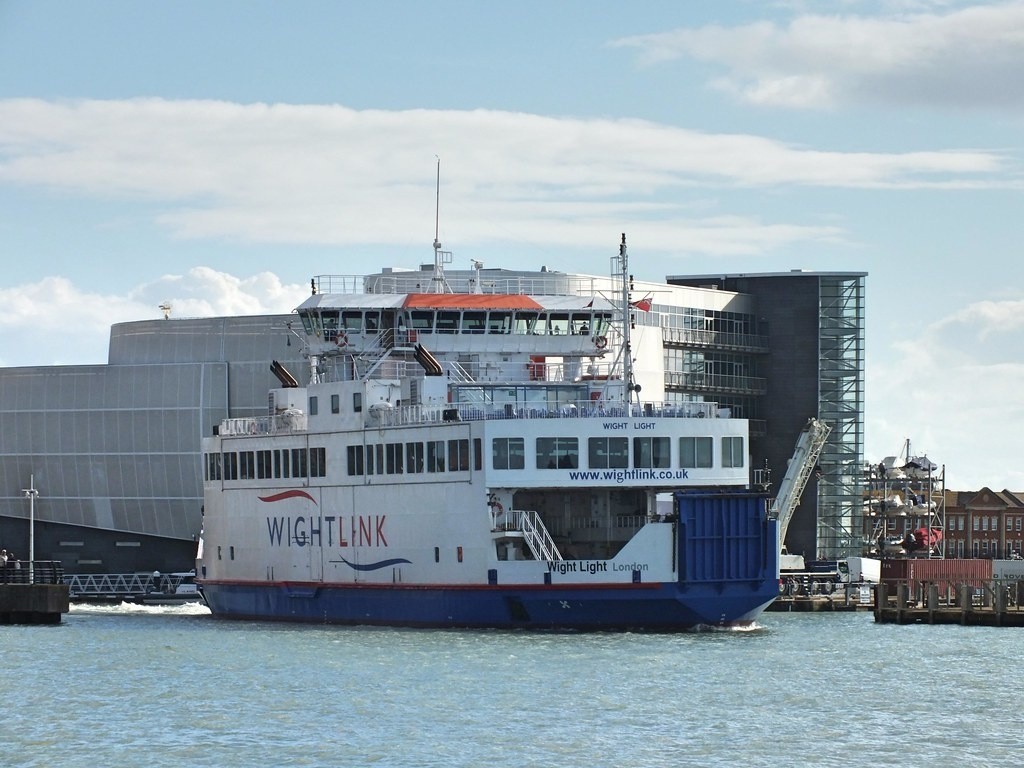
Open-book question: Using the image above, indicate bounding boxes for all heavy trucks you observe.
[777,556,852,596]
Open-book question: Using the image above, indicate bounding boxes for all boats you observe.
[194,149,783,638]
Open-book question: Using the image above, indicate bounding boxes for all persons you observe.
[578,322,589,335]
[6,552,17,583]
[327,319,336,334]
[0,549,8,583]
[367,320,375,332]
[547,455,574,468]
